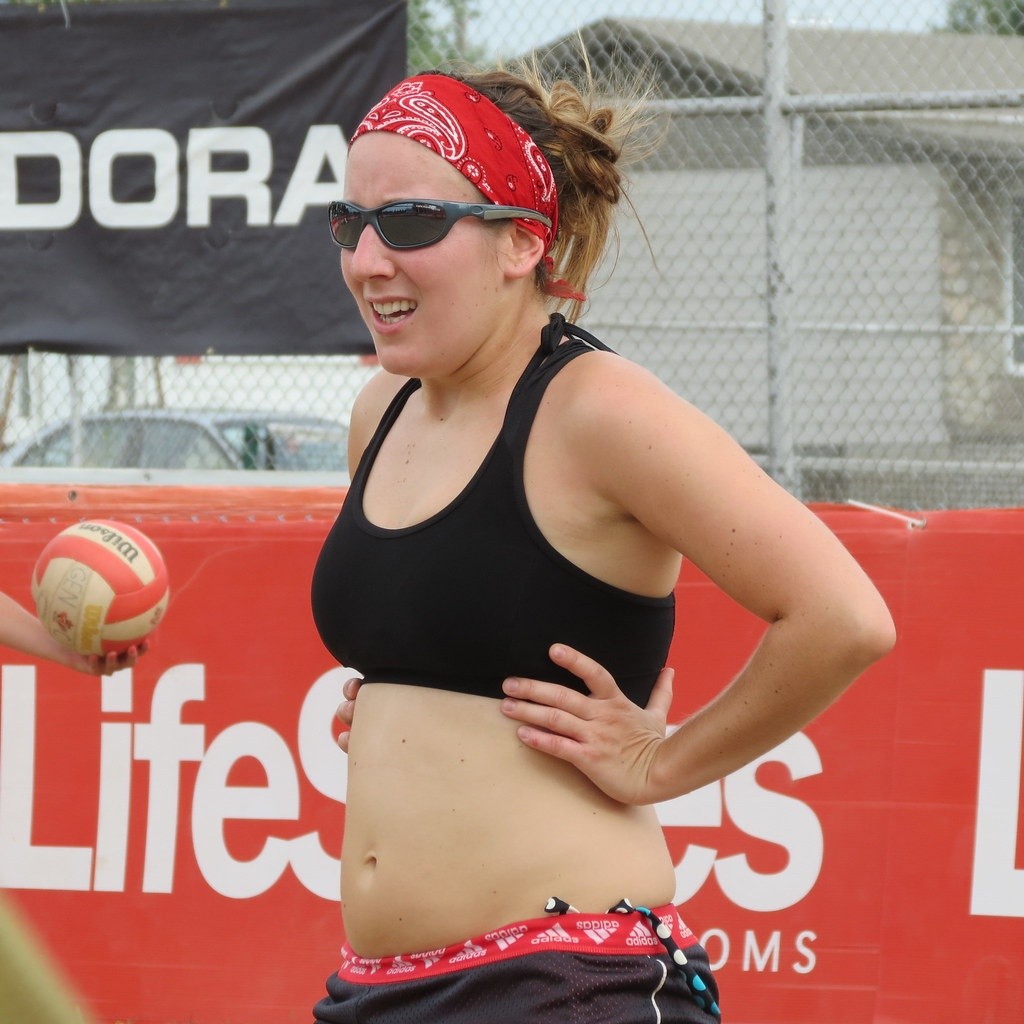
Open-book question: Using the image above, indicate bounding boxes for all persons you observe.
[309,68,898,1024]
[0,591,151,675]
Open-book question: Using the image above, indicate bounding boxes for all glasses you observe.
[327,200,553,251]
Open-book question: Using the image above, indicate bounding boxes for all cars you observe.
[0,406,353,489]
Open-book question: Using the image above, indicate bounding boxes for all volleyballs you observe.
[30,519,169,656]
